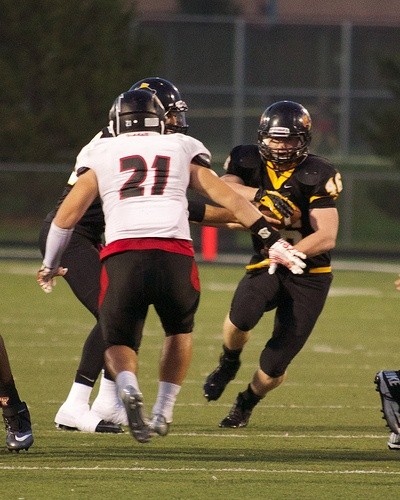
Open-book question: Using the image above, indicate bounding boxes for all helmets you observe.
[128,77,189,134]
[256,99,313,158]
[107,89,168,136]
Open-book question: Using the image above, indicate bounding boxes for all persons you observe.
[203,101,342,429]
[36,90,308,443]
[0,334,34,453]
[38,77,308,433]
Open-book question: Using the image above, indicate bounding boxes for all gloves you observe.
[267,238,307,275]
[35,263,69,294]
[254,186,302,221]
[244,257,273,270]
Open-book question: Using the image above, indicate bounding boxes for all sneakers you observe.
[202,352,241,402]
[90,377,130,426]
[217,391,254,427]
[386,431,400,449]
[118,385,154,442]
[2,401,35,453]
[54,380,125,434]
[148,413,171,437]
[373,369,400,437]
